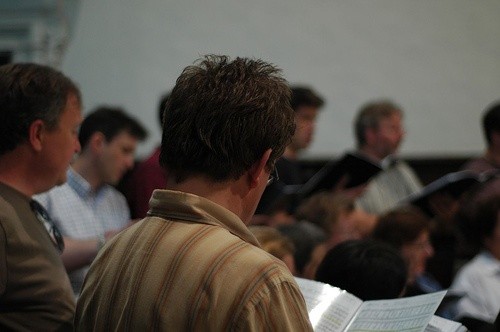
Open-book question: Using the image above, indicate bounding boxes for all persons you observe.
[74,53,316,331]
[0,62,82,331]
[244,84,500,332]
[32,93,178,304]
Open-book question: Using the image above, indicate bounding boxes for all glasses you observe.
[29,199,64,252]
[266,158,279,186]
